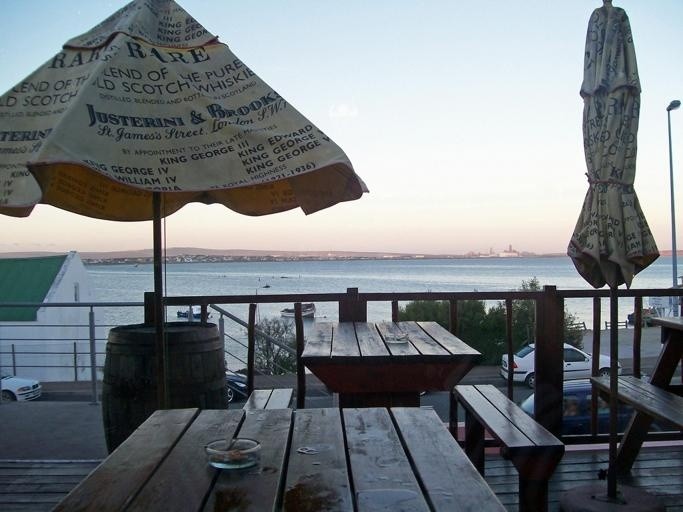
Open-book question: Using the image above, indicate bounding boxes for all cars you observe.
[499,343,622,390]
[499,379,664,460]
[0,370,42,402]
[224,371,248,404]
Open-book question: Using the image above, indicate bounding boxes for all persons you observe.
[566,400,580,418]
[597,398,609,414]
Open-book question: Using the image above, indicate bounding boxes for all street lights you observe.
[666,99,681,317]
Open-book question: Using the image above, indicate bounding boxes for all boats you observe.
[281,303,315,317]
[626,306,664,326]
[177,305,210,319]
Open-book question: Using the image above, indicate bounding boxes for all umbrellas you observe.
[0,0,370,409]
[565,1,659,496]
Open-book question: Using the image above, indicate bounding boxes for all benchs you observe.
[589,371,683,480]
[240,386,294,410]
[449,380,568,510]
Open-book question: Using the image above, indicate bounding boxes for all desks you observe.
[53,408,514,510]
[605,311,682,481]
[296,319,483,406]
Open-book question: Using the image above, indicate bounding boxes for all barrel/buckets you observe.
[99,320,230,456]
